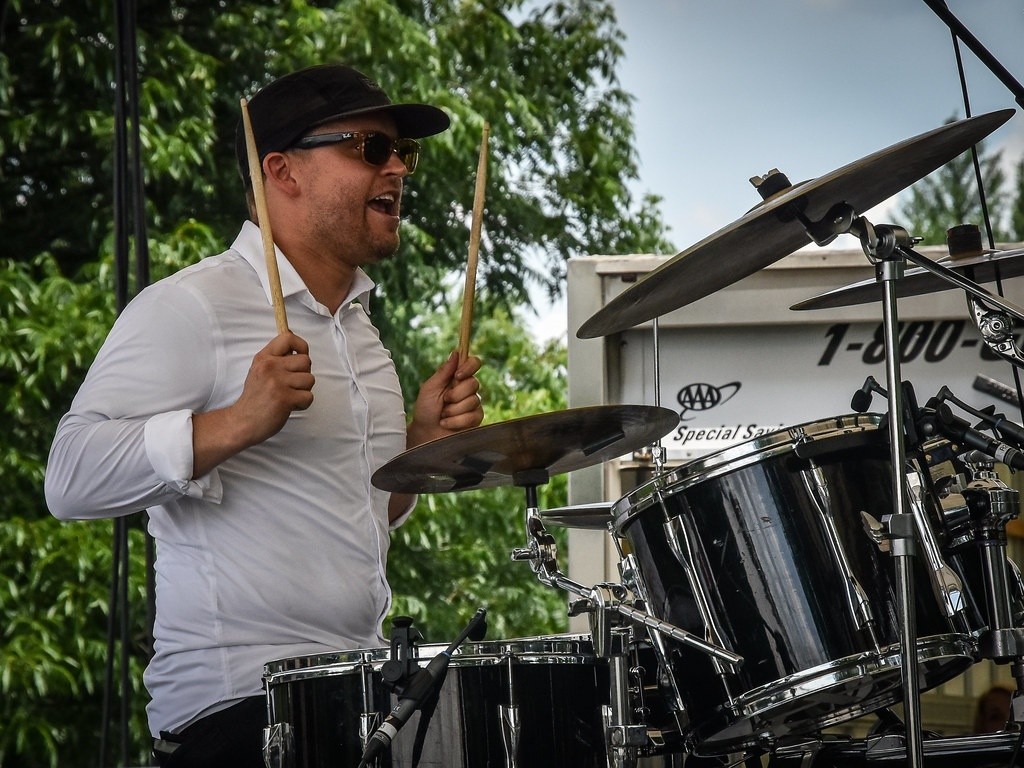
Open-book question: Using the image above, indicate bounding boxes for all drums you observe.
[931,503,1024,660]
[255,635,610,768]
[610,411,980,757]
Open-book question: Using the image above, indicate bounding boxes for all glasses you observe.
[286,131,420,175]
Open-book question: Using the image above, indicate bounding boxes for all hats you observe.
[235,64,452,190]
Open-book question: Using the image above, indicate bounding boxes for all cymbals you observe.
[788,246,1023,315]
[371,403,680,498]
[577,108,1017,344]
[533,501,617,531]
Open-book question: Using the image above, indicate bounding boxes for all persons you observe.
[44,66,484,768]
